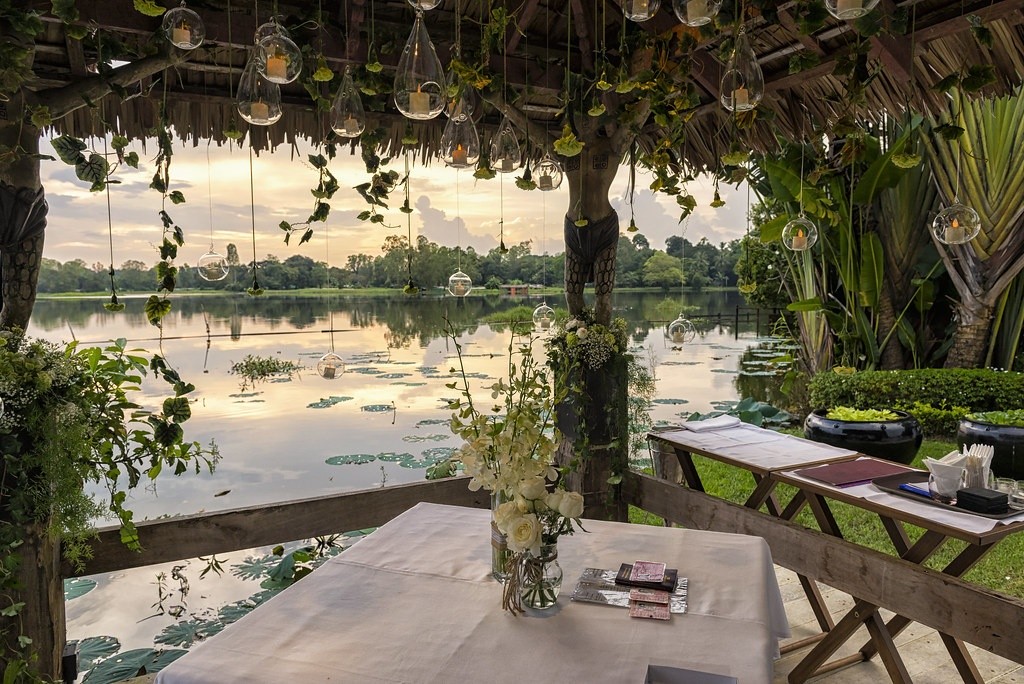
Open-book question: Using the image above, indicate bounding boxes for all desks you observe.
[645,421,1024,684]
[154,501,792,684]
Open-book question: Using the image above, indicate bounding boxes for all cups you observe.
[929,469,1024,511]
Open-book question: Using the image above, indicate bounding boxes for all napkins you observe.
[923,443,995,495]
[681,413,742,433]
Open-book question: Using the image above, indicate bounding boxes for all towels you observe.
[680,414,742,433]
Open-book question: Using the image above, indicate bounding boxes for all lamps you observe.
[316,34,345,379]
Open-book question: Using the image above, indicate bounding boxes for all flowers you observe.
[441,308,628,615]
[0,323,223,684]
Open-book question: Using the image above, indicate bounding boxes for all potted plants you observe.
[957,409,1024,481]
[804,406,923,466]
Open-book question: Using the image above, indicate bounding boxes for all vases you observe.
[490,521,517,586]
[519,545,563,610]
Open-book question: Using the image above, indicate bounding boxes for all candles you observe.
[172,20,191,50]
[836,0,863,19]
[944,217,965,243]
[631,0,649,22]
[731,82,749,112]
[539,170,552,191]
[454,281,466,298]
[502,160,513,173]
[344,111,360,138]
[408,82,430,115]
[250,53,287,126]
[686,0,711,27]
[792,227,808,252]
[450,144,469,168]
[447,95,463,117]
[672,326,684,345]
[540,316,552,331]
